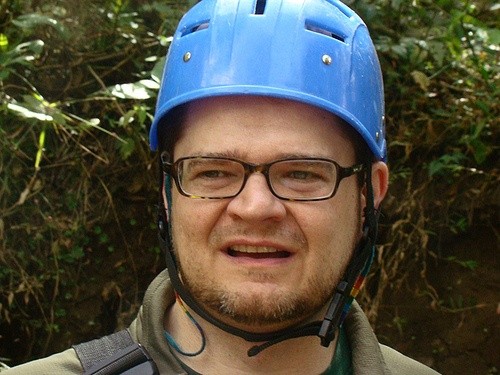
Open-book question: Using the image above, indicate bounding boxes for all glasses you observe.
[163,155,363,201]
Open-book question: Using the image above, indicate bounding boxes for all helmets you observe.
[149,0,387,159]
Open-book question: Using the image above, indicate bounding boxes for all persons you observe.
[0,0,442,375]
